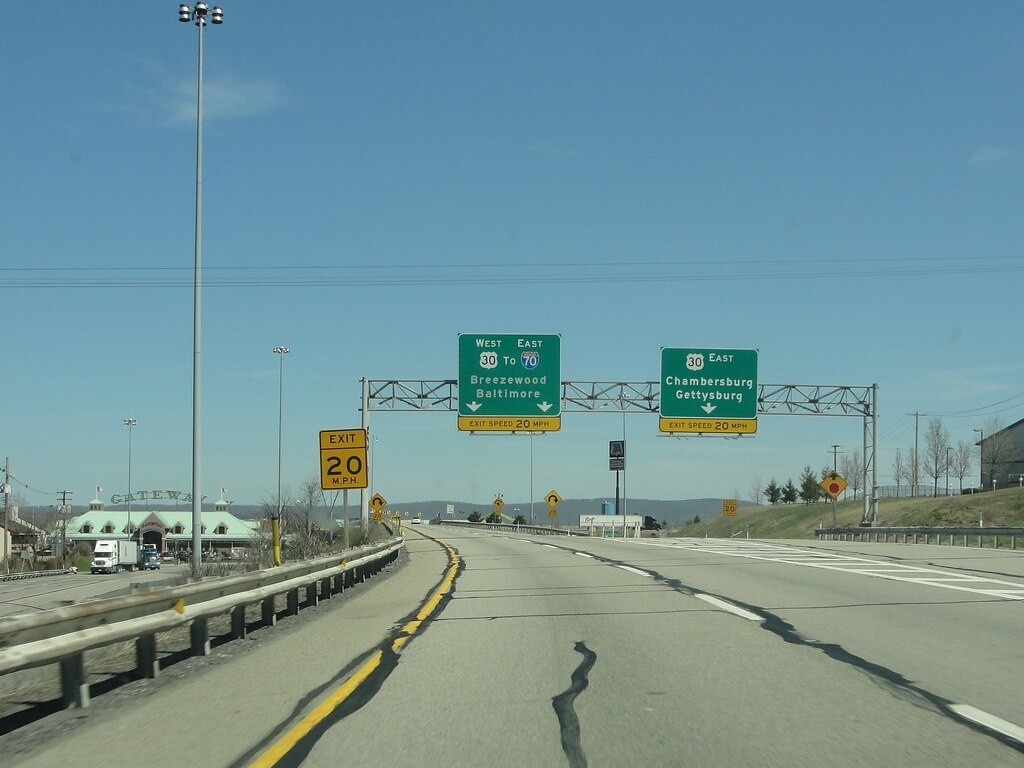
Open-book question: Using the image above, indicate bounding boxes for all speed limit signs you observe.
[722,498,737,516]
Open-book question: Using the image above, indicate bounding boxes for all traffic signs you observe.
[658,346,758,434]
[455,331,562,433]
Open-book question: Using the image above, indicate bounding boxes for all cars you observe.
[411,516,421,524]
[137,542,240,571]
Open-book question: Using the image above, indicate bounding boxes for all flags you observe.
[97,486,102,491]
[222,489,227,492]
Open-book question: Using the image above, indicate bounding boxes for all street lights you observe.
[946,447,954,496]
[273,345,291,529]
[974,429,983,492]
[123,418,139,542]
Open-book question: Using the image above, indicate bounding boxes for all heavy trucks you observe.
[91,539,138,575]
[579,513,664,535]
